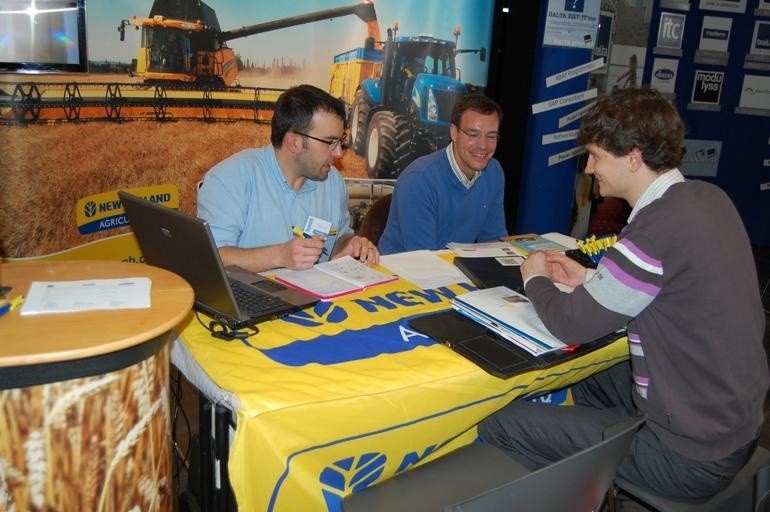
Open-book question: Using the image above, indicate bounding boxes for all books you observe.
[454,256,526,297]
[274,254,400,302]
[449,285,568,357]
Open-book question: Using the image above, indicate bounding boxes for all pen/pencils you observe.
[0,295,26,317]
[576,232,618,264]
[291,226,329,252]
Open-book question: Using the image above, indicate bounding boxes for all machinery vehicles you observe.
[330,22,486,183]
[116,0,378,91]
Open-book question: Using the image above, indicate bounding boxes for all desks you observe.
[170,232,584,512]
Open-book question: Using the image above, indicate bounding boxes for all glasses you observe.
[294,132,348,151]
[460,127,500,141]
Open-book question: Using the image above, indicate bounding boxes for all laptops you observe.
[116,188,321,330]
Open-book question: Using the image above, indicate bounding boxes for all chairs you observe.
[341,415,645,512]
[616,445,770,512]
[355,193,392,259]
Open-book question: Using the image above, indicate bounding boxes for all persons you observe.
[197,82,381,272]
[478,86,770,504]
[153,32,177,65]
[618,54,637,88]
[376,93,510,253]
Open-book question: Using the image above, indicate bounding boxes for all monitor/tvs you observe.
[0,0,90,76]
